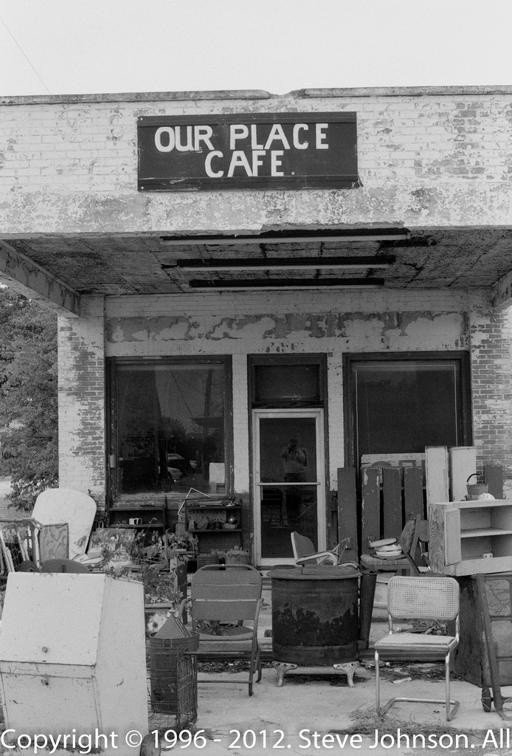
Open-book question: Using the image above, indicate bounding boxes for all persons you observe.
[281,437,308,529]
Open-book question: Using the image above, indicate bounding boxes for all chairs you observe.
[289,532,361,571]
[371,574,465,723]
[189,562,262,701]
[360,512,421,575]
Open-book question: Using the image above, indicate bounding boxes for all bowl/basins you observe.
[376,545,402,558]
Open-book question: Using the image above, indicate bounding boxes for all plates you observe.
[368,537,397,549]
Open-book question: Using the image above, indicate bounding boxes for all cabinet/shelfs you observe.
[427,499,512,578]
[166,492,243,572]
[107,506,166,548]
